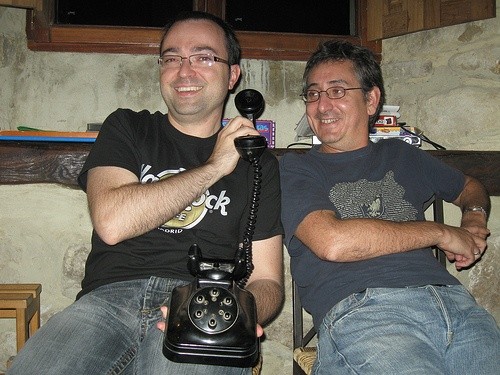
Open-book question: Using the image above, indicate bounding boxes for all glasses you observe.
[299,86,362,102]
[157,53,230,69]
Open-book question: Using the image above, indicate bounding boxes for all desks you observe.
[0,130,500,197]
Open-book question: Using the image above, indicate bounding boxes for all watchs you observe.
[461,205,487,219]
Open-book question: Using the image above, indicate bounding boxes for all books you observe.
[293,105,421,149]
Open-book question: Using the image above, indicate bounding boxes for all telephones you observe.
[233,89,266,163]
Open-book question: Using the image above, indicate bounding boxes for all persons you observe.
[4,12,284,375]
[281,38,500,375]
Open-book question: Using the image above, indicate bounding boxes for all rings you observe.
[473,249,480,255]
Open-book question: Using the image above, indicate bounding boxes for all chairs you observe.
[293,198,446,375]
[0,283,42,355]
